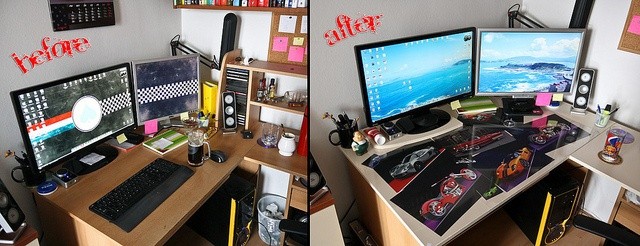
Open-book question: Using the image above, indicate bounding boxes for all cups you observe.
[594,109,611,128]
[328,121,358,148]
[187,133,211,167]
[261,123,280,146]
[601,127,628,164]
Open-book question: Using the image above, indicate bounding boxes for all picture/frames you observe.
[616,0,640,56]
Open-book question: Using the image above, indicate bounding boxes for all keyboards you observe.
[90,158,195,232]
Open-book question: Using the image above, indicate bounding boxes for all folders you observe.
[200,81,217,121]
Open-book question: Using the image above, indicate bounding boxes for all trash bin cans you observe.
[257,194,287,246]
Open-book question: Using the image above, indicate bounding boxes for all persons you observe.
[350,131,369,156]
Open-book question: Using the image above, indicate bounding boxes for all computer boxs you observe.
[185,166,257,246]
[504,159,591,246]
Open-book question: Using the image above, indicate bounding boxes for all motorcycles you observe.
[421,168,477,221]
[532,122,571,145]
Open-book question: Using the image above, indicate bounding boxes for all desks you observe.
[332,95,640,246]
[0,221,40,246]
[310,184,346,246]
[24,117,307,246]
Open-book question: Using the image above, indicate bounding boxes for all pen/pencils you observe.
[199,112,210,129]
[598,103,619,125]
[14,151,30,168]
[330,112,360,130]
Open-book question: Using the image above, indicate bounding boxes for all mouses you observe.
[205,150,227,163]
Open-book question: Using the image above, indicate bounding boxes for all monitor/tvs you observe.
[132,52,201,137]
[354,27,476,134]
[8,62,137,175]
[474,28,587,116]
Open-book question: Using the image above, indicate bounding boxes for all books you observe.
[143,129,188,155]
[173,0,307,8]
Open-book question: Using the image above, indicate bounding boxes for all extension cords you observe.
[349,219,378,246]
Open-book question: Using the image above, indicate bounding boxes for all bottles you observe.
[256,80,264,97]
[268,78,275,98]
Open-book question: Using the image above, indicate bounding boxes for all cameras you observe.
[381,121,402,141]
[54,169,79,187]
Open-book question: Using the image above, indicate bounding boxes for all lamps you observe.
[170,11,237,72]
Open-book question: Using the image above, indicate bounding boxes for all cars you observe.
[496,147,531,180]
[453,131,501,152]
[390,147,436,179]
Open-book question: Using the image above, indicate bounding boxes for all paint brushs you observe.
[280,124,286,137]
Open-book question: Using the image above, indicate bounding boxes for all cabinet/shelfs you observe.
[171,0,307,134]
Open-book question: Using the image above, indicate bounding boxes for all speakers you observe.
[0,177,26,244]
[309,151,329,205]
[571,68,598,117]
[221,92,238,134]
